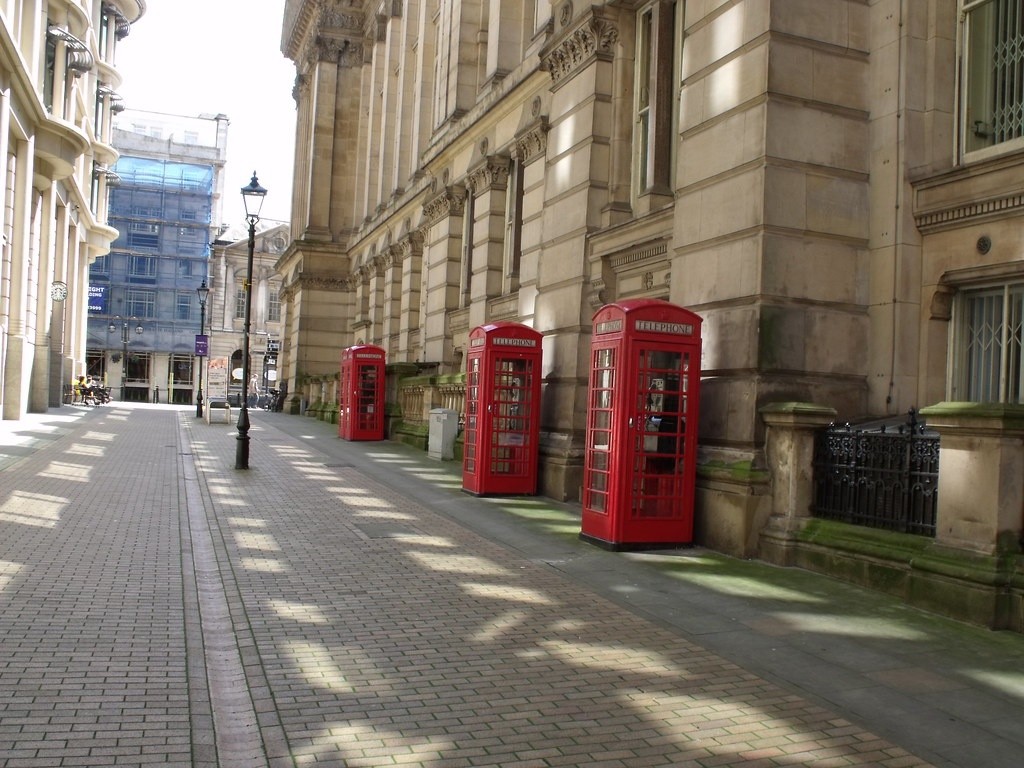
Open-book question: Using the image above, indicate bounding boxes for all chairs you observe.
[62,383,112,407]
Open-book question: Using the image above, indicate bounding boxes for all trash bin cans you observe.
[427,408,460,460]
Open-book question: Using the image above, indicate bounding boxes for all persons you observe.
[77,375,113,404]
[245,373,259,409]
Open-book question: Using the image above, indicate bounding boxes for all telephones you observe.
[646,378,680,418]
[509,378,524,405]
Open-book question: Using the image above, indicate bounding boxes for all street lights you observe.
[195,279,211,418]
[108,314,144,402]
[236,169,269,471]
